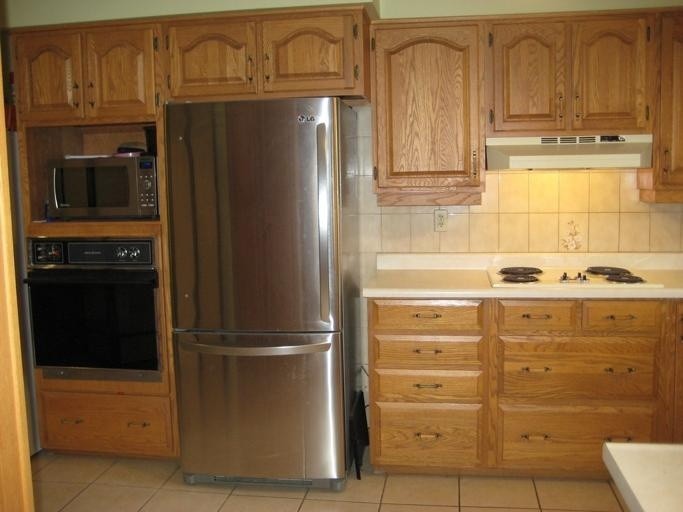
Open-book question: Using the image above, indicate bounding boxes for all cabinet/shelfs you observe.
[485,7,648,130]
[367,293,491,473]
[160,5,370,101]
[634,3,683,204]
[21,122,176,460]
[8,15,162,123]
[368,16,484,207]
[497,298,682,481]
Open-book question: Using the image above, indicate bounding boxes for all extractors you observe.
[485,134,654,173]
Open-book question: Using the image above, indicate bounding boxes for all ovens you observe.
[22,237,164,381]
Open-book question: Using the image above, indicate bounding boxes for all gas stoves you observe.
[485,264,663,289]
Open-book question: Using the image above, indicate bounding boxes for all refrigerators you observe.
[161,95,366,490]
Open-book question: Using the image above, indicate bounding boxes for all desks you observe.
[594,441,681,512]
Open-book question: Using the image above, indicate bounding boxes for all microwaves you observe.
[45,152,158,219]
